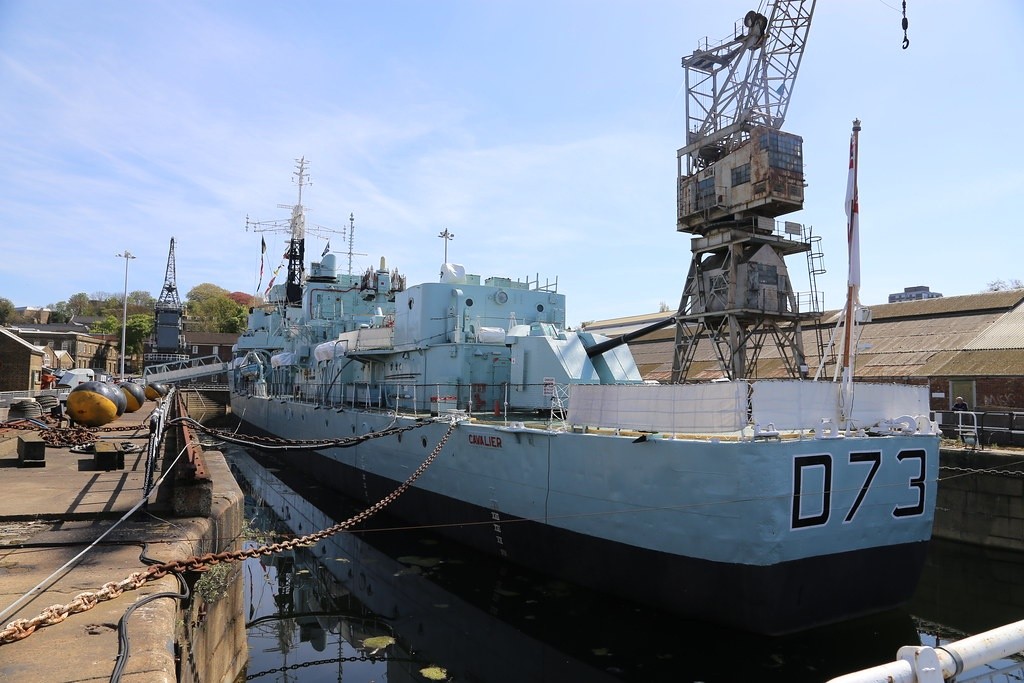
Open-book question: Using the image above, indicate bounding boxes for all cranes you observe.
[667,0,913,386]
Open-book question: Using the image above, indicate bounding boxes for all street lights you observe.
[114,249,136,382]
[438,228,454,267]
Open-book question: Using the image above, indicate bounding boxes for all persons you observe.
[951,398,971,446]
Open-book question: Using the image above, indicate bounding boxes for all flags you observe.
[845,135,860,292]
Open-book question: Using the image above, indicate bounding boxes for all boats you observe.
[225,1,944,683]
